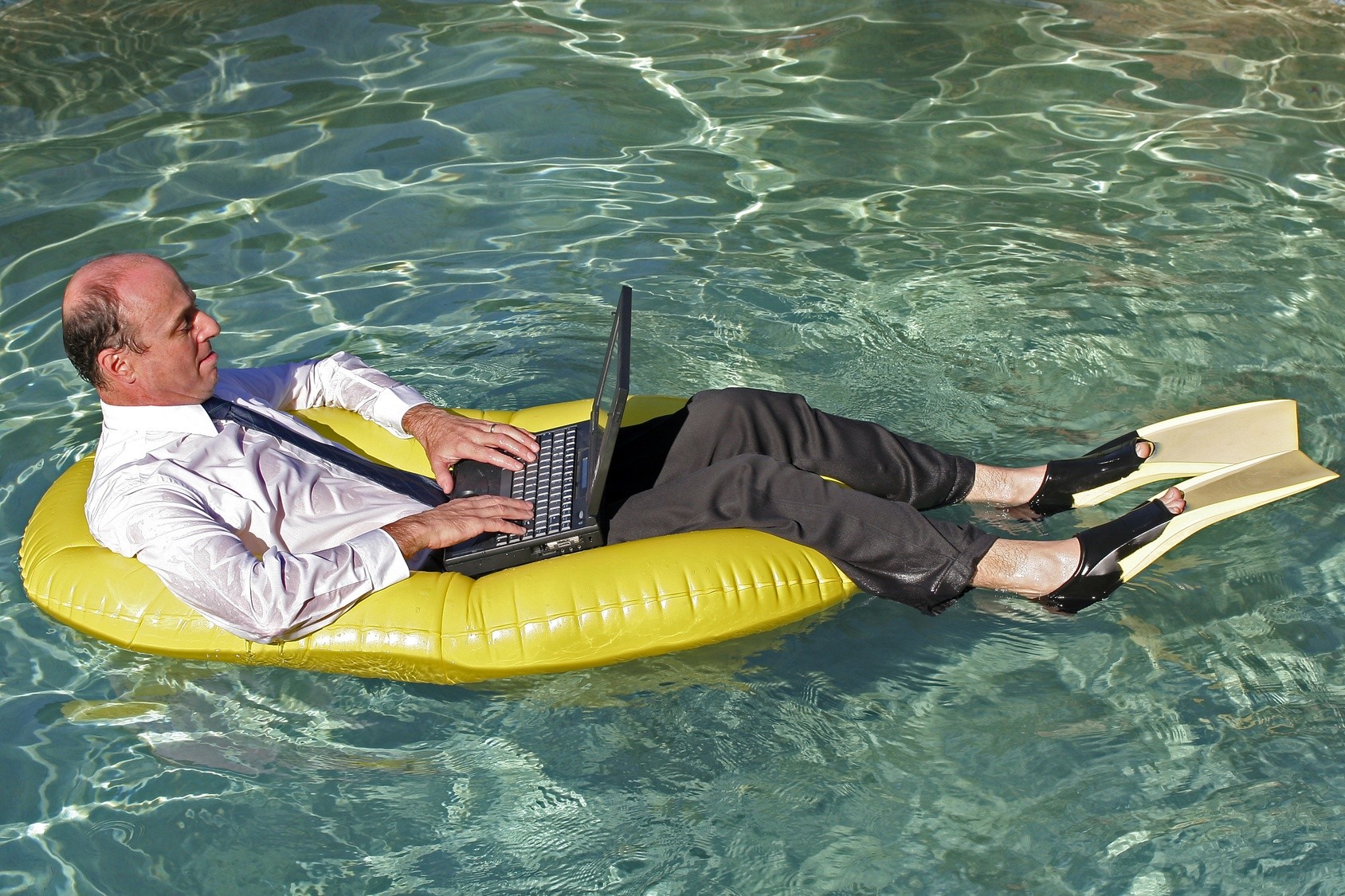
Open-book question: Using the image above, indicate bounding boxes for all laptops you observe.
[442,287,633,576]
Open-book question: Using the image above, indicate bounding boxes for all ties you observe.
[200,397,450,508]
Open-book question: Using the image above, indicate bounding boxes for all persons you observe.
[62,252,1189,645]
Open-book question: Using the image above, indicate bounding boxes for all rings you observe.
[491,423,497,432]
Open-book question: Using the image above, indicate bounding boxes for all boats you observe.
[16,395,861,682]
